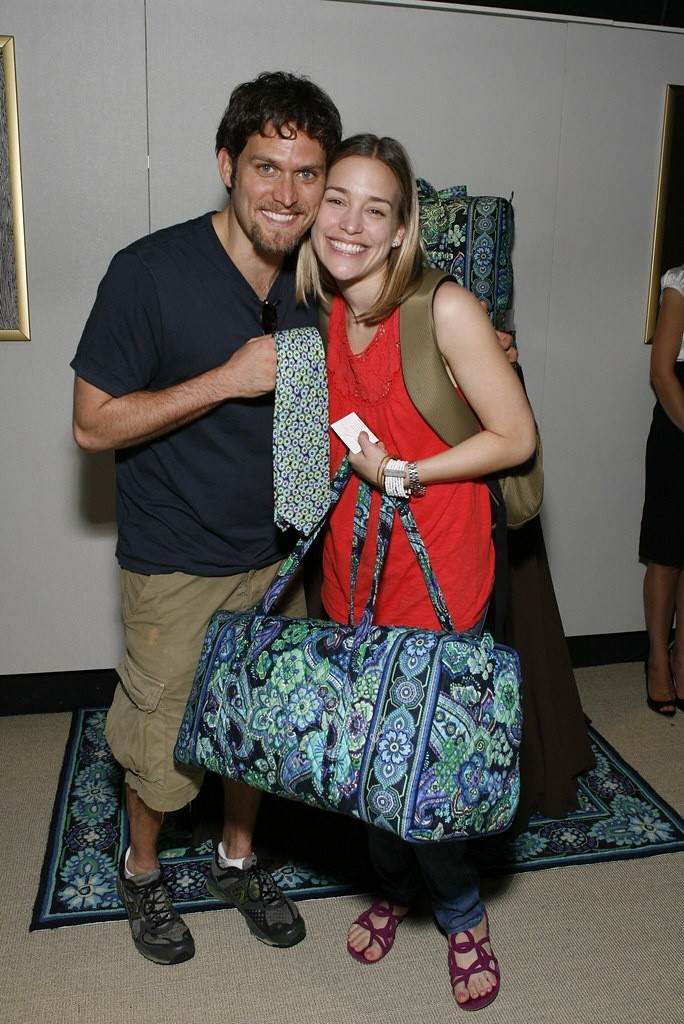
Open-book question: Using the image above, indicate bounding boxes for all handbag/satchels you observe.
[415,178,515,328]
[173,455,524,846]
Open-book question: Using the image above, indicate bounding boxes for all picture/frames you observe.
[0,35,32,341]
[645,84,684,344]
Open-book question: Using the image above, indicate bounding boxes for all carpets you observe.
[27,707,684,932]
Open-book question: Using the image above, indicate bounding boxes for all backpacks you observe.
[319,268,545,529]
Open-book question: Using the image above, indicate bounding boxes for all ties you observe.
[271,326,331,538]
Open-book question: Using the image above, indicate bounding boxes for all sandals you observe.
[447,910,501,1011]
[346,896,410,965]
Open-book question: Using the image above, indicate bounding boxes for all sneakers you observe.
[206,847,306,948]
[116,845,195,965]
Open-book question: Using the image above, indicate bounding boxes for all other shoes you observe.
[644,649,677,718]
[668,640,684,712]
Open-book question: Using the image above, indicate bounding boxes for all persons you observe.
[295,134,538,1012]
[65,71,519,966]
[635,265,684,718]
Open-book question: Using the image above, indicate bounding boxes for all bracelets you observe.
[376,453,427,499]
